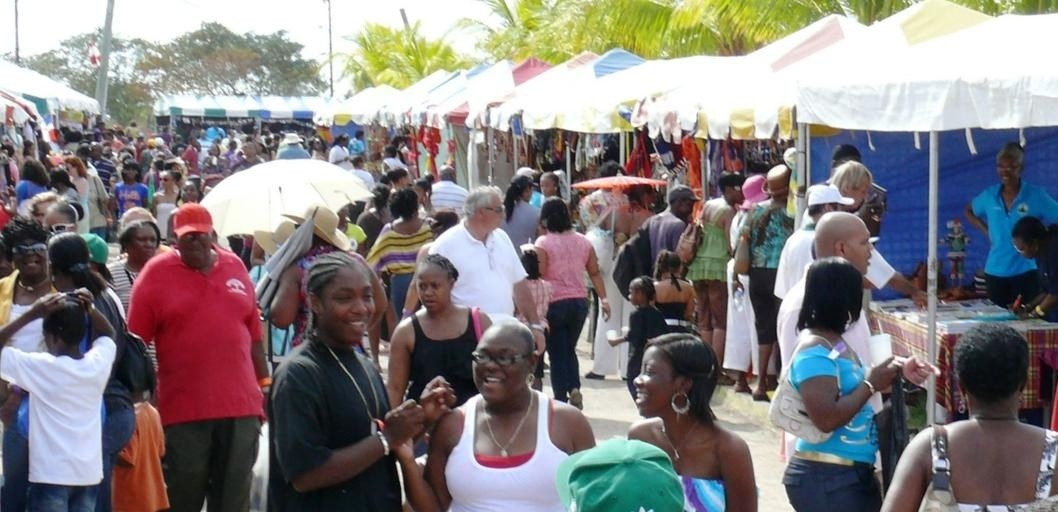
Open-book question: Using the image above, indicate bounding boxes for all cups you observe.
[867,331,894,367]
[606,329,617,341]
[620,327,630,337]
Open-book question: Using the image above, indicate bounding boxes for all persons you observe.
[46,232,137,509]
[119,201,271,510]
[1,287,119,510]
[626,329,760,512]
[374,315,599,510]
[267,249,459,511]
[770,255,900,512]
[0,117,1058,411]
[879,320,1058,511]
[111,393,173,510]
[775,209,943,501]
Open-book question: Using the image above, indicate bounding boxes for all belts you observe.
[791,450,854,465]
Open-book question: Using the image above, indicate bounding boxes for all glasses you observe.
[48,224,73,235]
[12,244,47,258]
[470,351,532,366]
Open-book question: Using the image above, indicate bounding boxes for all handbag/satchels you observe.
[768,381,834,444]
[120,332,156,393]
[677,220,703,265]
[612,228,651,302]
[875,361,907,497]
[917,479,958,511]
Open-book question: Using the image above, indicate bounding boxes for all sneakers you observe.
[569,390,583,412]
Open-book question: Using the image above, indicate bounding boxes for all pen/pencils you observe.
[1013,294,1023,312]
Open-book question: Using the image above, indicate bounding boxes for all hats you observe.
[808,183,855,206]
[282,134,304,147]
[511,175,539,189]
[147,137,164,148]
[80,233,107,265]
[669,185,702,203]
[738,164,793,210]
[119,202,351,255]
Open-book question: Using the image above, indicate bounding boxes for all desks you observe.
[866,298,1057,442]
